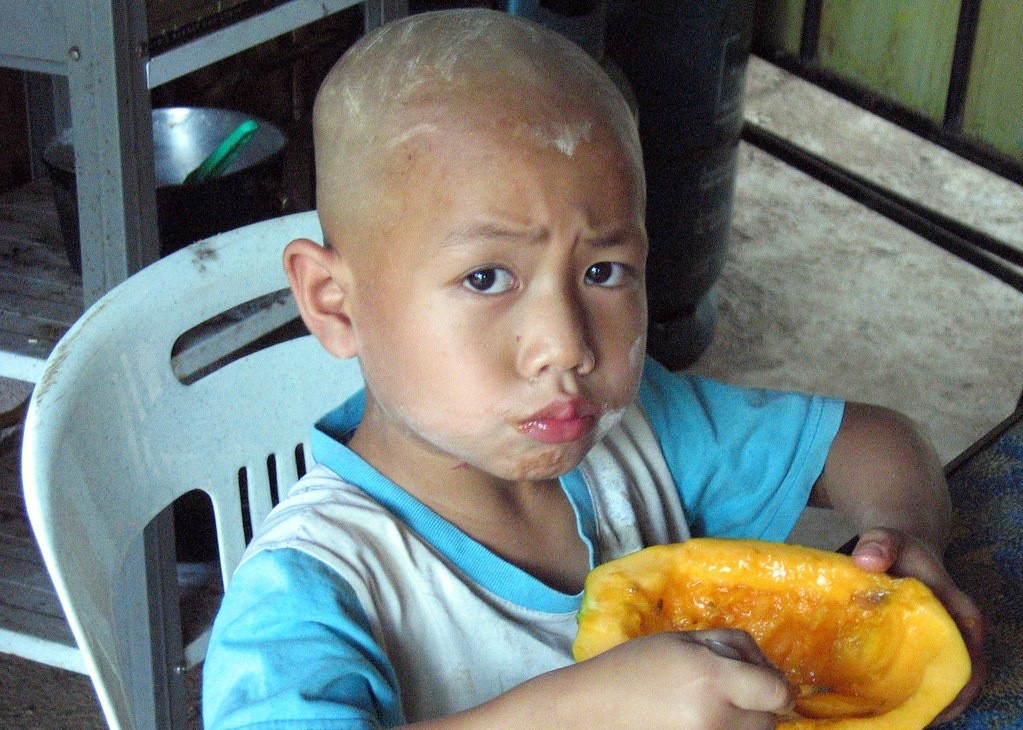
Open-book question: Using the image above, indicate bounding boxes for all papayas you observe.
[572,538,973,730]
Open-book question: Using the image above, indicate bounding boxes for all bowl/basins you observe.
[33,106,291,281]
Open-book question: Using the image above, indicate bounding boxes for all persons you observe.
[204,6,990,728]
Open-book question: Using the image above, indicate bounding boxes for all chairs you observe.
[20,209,367,730]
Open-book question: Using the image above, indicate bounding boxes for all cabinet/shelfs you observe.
[0,0,407,730]
